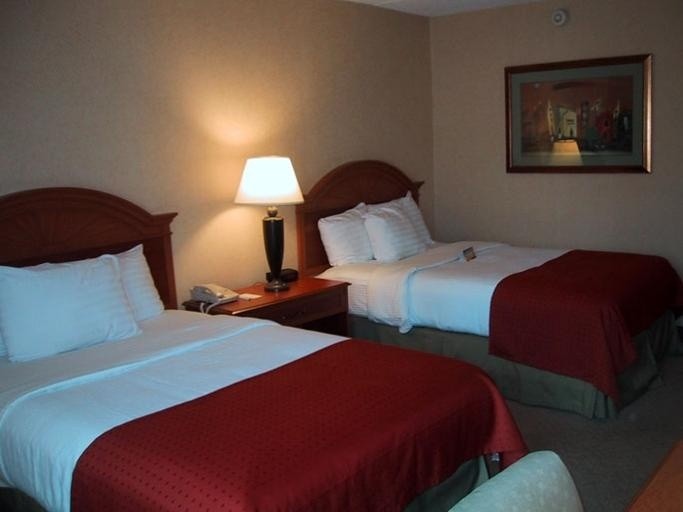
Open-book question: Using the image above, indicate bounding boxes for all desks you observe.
[621,436,683,512]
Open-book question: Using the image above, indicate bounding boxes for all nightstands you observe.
[183,277,351,340]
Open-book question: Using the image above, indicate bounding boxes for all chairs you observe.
[450,450,584,511]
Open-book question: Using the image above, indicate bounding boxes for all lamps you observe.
[234,154,305,294]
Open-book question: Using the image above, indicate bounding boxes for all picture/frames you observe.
[504,52,654,176]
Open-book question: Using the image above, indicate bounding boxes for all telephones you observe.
[190,284,239,304]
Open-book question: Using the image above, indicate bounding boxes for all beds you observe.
[0,187,528,512]
[296,159,683,420]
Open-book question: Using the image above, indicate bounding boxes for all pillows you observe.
[119,243,166,324]
[316,190,435,267]
[0,255,140,362]
